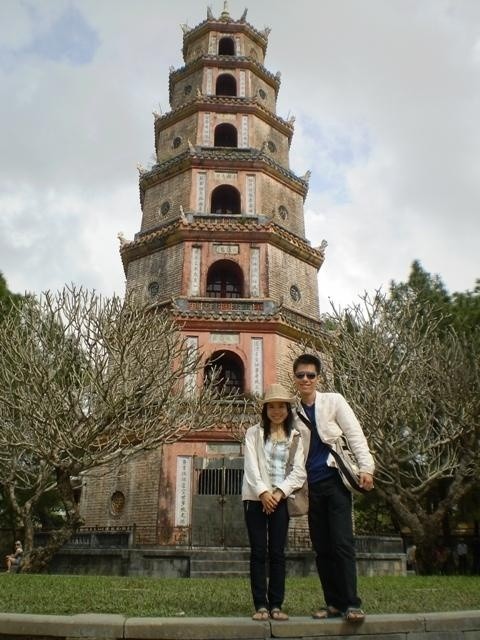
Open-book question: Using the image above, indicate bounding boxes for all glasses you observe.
[294,371,316,379]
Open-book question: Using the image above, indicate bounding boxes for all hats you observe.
[256,383,296,406]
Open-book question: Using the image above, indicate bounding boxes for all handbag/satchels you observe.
[336,435,375,496]
[287,486,310,516]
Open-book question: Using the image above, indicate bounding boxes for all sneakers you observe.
[311,607,344,619]
[344,607,365,622]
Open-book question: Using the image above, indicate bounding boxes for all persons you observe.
[287,354,379,622]
[405,540,417,571]
[241,383,306,621]
[4,539,23,573]
[433,540,450,576]
[456,538,470,575]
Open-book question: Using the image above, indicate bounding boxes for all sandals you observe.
[270,608,288,620]
[252,608,269,621]
